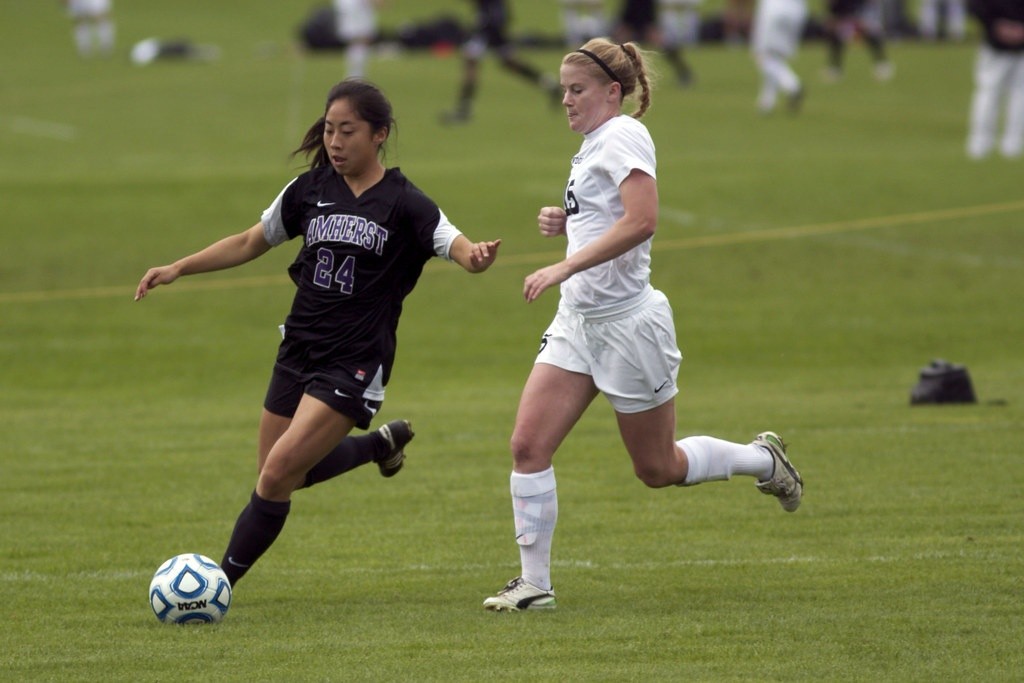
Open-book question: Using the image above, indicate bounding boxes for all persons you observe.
[482,36,803,613]
[60,1,1024,161]
[134,75,500,587]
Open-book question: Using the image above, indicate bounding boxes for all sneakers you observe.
[754,431,806,513]
[373,419,415,478]
[483,578,555,613]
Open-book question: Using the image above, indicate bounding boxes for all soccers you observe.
[149,553,232,624]
